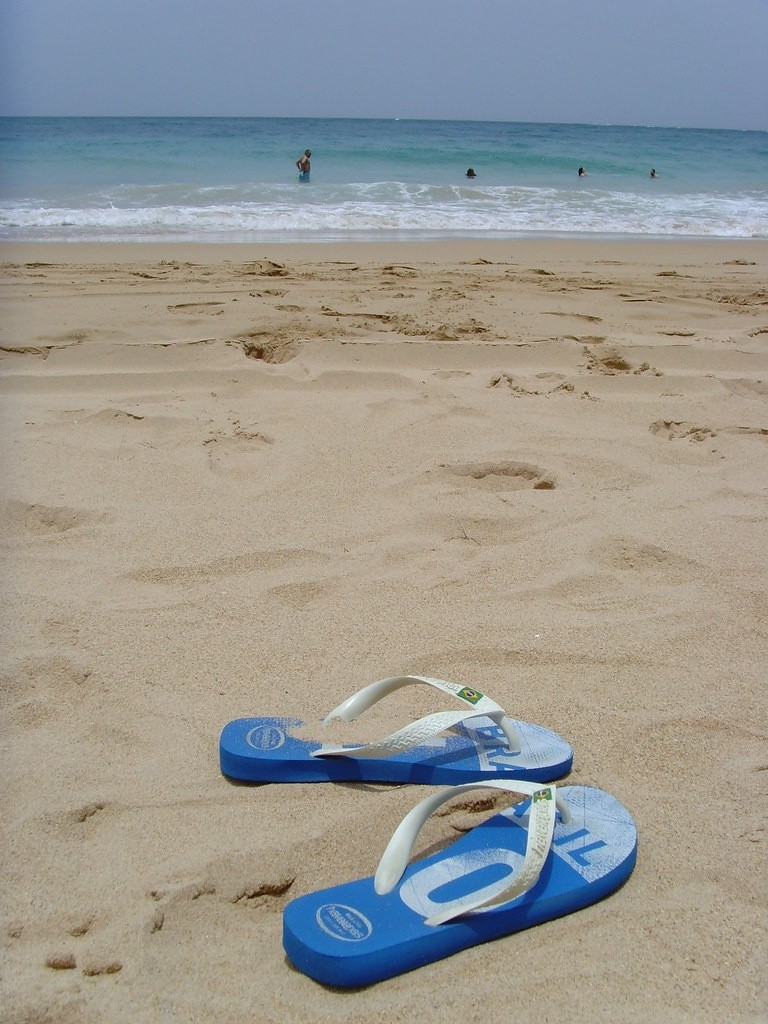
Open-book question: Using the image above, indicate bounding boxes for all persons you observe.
[296,149,311,182]
[579,167,587,176]
[466,168,474,175]
[651,169,655,176]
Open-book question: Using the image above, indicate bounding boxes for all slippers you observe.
[282,779,638,988]
[220,675,573,786]
